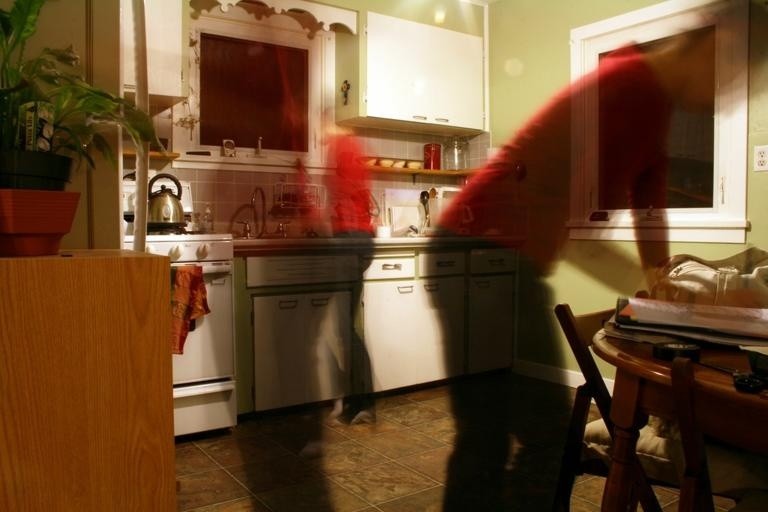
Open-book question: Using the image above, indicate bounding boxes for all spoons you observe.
[420,191,429,220]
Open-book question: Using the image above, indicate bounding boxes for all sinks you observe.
[240,231,286,241]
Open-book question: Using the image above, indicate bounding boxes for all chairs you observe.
[551,291,764,512]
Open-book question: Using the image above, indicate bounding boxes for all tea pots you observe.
[147,173,187,226]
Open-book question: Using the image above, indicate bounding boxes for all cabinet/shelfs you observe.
[368,165,473,184]
[335,12,492,141]
[1,247,178,511]
[467,248,521,374]
[356,244,468,398]
[235,247,360,419]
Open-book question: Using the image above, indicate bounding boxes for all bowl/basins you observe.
[392,160,406,169]
[357,158,378,167]
[407,161,421,170]
[378,158,394,168]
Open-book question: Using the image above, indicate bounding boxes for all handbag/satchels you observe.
[669,248,768,309]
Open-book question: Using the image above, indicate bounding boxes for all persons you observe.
[181,0,378,512]
[296,106,377,428]
[426,0,768,512]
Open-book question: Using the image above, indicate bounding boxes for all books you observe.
[598,292,767,357]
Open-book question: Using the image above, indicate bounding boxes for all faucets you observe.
[245,187,267,239]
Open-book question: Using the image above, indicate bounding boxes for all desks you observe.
[594,329,768,512]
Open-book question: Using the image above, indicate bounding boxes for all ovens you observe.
[124,241,236,386]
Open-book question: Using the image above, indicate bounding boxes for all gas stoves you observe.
[122,180,233,242]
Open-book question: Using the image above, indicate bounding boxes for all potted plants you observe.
[0,0,170,259]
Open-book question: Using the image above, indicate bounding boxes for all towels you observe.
[171,265,210,355]
